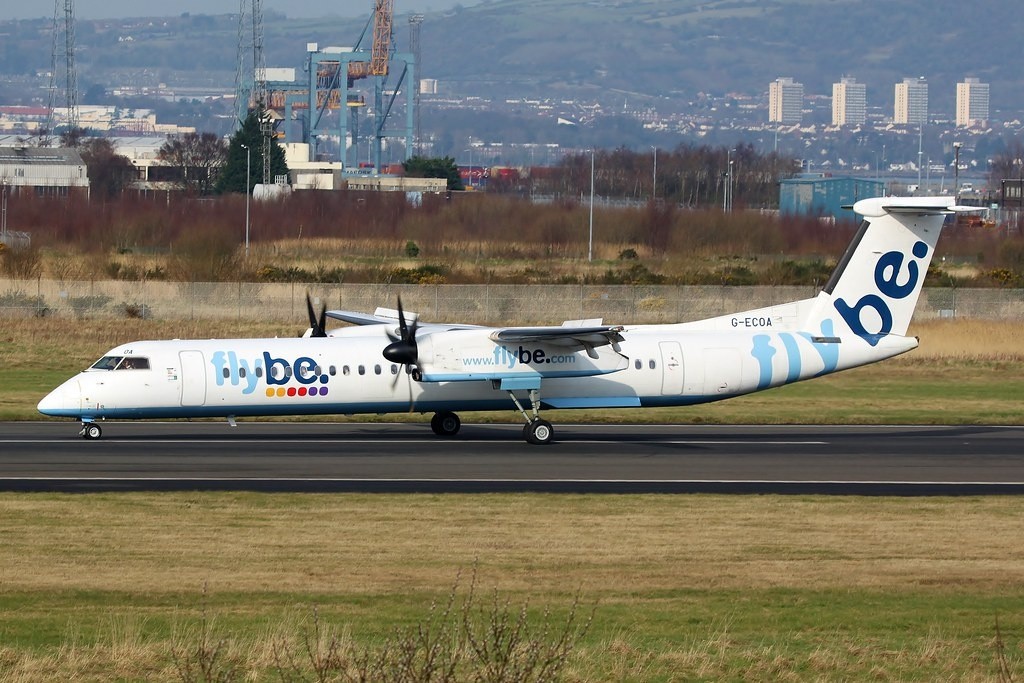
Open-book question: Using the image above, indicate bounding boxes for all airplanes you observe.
[36,194,991,446]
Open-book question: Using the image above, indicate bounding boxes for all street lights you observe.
[587,149,594,262]
[723,149,737,212]
[241,144,251,252]
[872,150,878,197]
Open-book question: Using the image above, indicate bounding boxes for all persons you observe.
[115,359,134,369]
